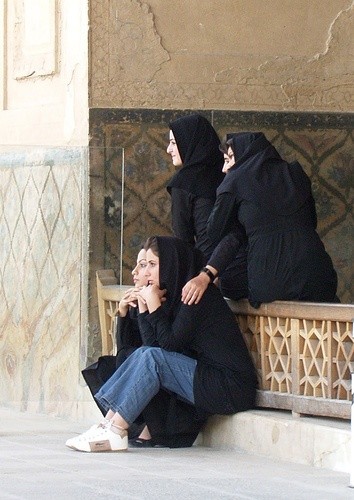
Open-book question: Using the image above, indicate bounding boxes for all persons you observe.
[180,131,341,309]
[65,236,258,452]
[82,238,209,448]
[166,113,250,300]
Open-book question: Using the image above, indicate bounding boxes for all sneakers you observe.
[66,419,128,451]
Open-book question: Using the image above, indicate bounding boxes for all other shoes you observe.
[129,434,154,447]
